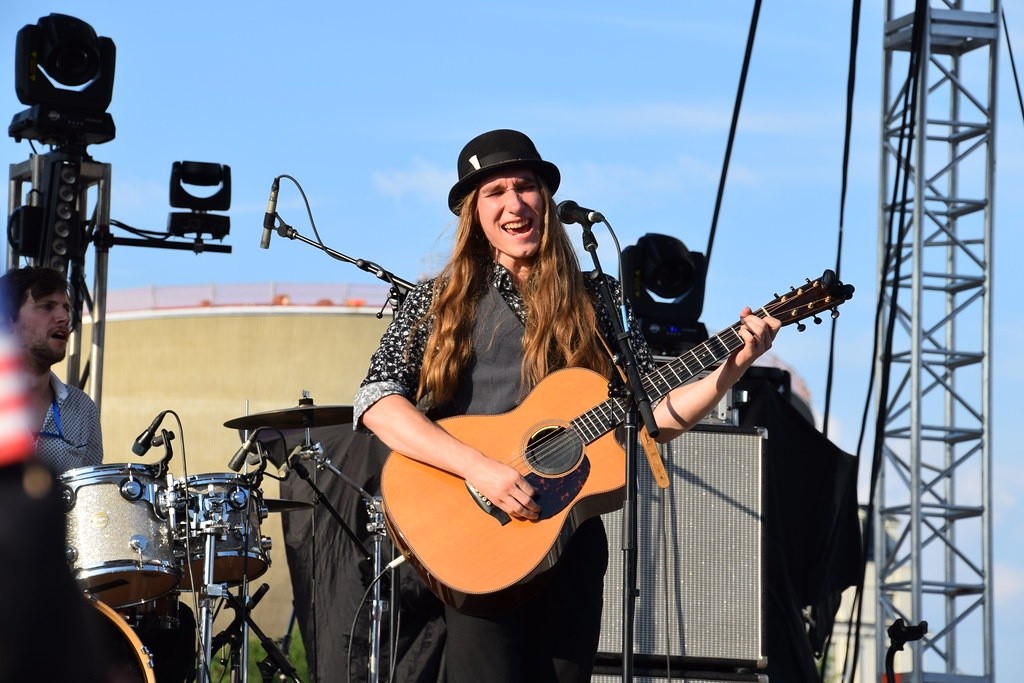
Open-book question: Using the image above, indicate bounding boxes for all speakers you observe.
[593,424,768,668]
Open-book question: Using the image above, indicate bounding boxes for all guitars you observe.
[378,270,856,615]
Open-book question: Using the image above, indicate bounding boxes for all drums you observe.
[81,593,195,682]
[165,472,270,590]
[60,462,185,610]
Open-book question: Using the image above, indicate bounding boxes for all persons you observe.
[352,129,780,683]
[0,265,127,683]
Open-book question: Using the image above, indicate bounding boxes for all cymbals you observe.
[223,405,353,430]
[264,499,316,512]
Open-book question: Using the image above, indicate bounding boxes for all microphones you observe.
[261,178,279,248]
[133,411,166,456]
[228,429,259,472]
[277,445,303,481]
[556,200,605,224]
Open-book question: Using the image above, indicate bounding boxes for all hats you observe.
[619,233,705,328]
[447,129,561,218]
